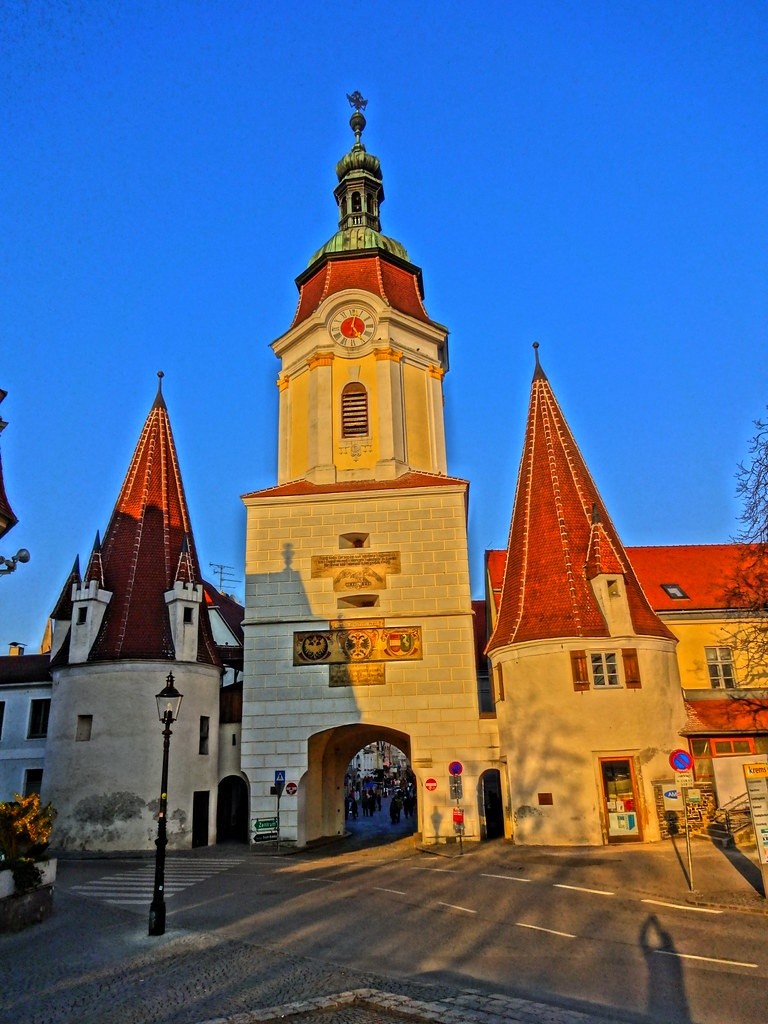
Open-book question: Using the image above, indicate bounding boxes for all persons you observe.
[362,788,382,817]
[389,790,415,825]
[345,790,358,820]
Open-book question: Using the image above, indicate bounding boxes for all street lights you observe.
[149,669,185,936]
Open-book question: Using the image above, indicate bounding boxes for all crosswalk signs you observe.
[275,770,286,784]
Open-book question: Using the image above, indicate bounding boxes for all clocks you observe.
[330,308,374,347]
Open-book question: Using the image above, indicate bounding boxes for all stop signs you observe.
[425,778,438,791]
